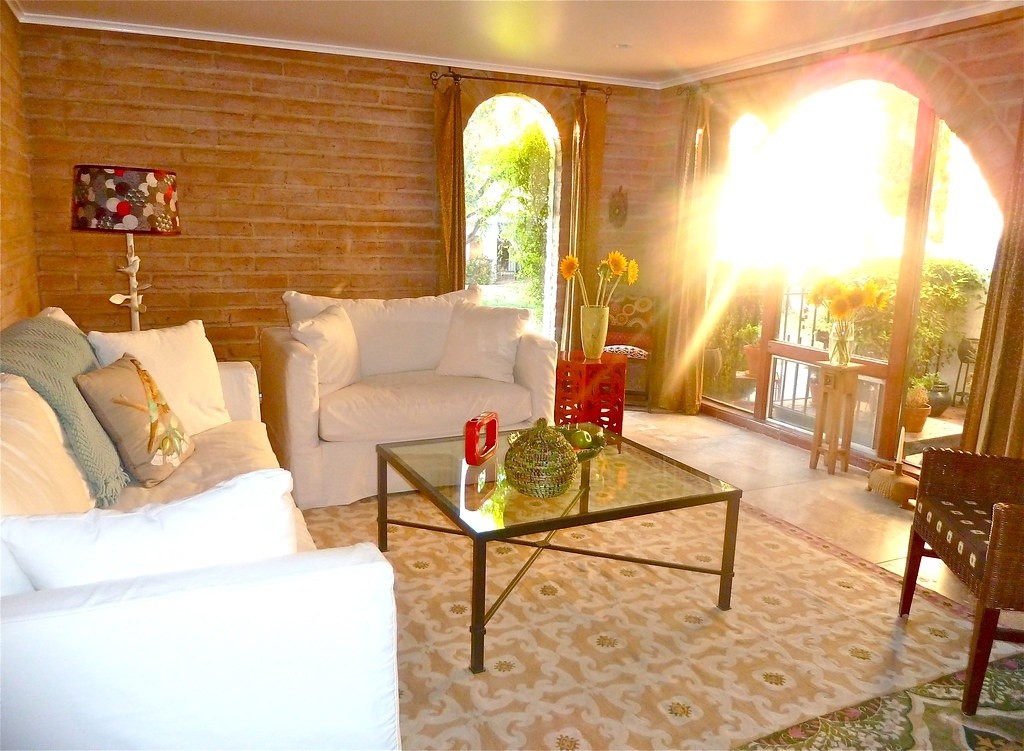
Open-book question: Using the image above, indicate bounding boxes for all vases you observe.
[828,321,855,366]
[958,337,979,362]
[579,304,610,358]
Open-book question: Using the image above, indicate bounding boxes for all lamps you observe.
[71,162,184,331]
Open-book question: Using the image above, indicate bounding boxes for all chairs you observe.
[581,291,660,414]
[898,448,1023,714]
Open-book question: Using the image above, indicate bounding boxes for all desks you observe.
[809,360,866,475]
[735,370,781,415]
[774,398,964,461]
[953,361,976,406]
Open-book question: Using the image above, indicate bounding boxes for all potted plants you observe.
[703,296,721,380]
[810,371,820,408]
[903,371,953,434]
[734,322,763,377]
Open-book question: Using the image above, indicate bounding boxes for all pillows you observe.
[88,318,232,436]
[291,303,360,398]
[435,296,530,383]
[0,469,301,591]
[76,351,195,490]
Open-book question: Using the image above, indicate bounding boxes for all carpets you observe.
[299,444,1024,751]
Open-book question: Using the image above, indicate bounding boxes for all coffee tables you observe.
[376,421,744,674]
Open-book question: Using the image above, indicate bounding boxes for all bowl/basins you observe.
[508,423,606,462]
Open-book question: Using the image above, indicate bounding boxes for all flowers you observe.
[557,249,639,308]
[806,274,891,363]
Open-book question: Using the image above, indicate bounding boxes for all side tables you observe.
[554,350,627,455]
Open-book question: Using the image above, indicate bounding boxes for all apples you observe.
[569,430,592,449]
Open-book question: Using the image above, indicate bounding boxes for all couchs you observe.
[0,304,401,751]
[258,282,558,510]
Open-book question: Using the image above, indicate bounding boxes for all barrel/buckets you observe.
[958,338,980,363]
